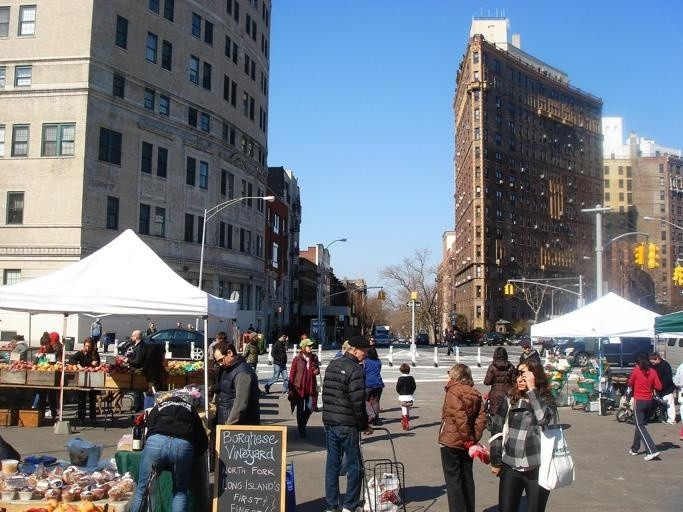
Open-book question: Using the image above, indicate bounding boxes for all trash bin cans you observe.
[65,337,74,351]
[108,333,115,344]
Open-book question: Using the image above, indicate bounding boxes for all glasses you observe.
[218,353,227,363]
[515,370,526,376]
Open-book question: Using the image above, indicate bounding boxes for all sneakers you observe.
[324,505,364,512]
[664,421,676,425]
[368,416,374,422]
[299,430,306,437]
[644,451,660,460]
[629,448,638,455]
[265,384,270,393]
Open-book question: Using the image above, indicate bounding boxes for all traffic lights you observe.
[671,268,683,286]
[378,290,385,300]
[648,243,660,270]
[634,242,645,265]
[504,284,513,294]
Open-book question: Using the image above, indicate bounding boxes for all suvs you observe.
[556,337,654,367]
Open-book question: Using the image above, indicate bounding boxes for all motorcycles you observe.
[615,394,657,423]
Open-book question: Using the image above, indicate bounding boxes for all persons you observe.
[175,321,183,330]
[31,330,61,418]
[122,330,147,412]
[439,364,486,511]
[649,354,677,426]
[104,333,110,352]
[207,323,264,403]
[627,353,663,461]
[488,358,557,512]
[69,337,100,421]
[319,335,373,512]
[129,396,207,512]
[146,322,157,336]
[519,337,541,362]
[186,323,195,332]
[672,362,683,440]
[485,346,515,412]
[265,333,290,397]
[311,354,321,412]
[396,363,416,430]
[10,336,28,362]
[335,339,384,425]
[446,329,461,355]
[287,339,319,437]
[91,318,102,346]
[213,341,259,425]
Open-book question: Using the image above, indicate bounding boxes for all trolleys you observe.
[359,425,408,511]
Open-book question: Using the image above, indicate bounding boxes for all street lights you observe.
[318,238,346,345]
[194,195,275,333]
[594,232,649,299]
[642,217,683,233]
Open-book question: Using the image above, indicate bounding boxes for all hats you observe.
[521,339,531,347]
[300,339,314,347]
[348,336,371,349]
[249,332,258,339]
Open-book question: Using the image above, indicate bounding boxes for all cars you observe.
[116,328,209,362]
[373,333,551,346]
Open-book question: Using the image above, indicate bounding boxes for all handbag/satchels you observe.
[538,428,576,491]
[315,386,324,411]
[650,397,670,420]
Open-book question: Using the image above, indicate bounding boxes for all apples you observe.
[6,360,31,371]
[31,362,62,372]
[167,360,205,376]
[101,358,129,373]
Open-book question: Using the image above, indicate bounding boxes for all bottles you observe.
[132,410,148,451]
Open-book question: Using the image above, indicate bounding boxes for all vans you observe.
[665,336,683,374]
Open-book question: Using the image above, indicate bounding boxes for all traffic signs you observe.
[405,301,422,308]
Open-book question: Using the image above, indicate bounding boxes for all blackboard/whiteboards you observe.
[212,424,287,512]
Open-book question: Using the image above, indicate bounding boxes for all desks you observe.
[115,450,208,512]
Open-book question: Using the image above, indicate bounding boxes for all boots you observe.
[401,415,410,430]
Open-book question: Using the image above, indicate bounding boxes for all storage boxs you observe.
[0,368,216,389]
[588,400,600,412]
[0,408,12,425]
[17,410,40,427]
